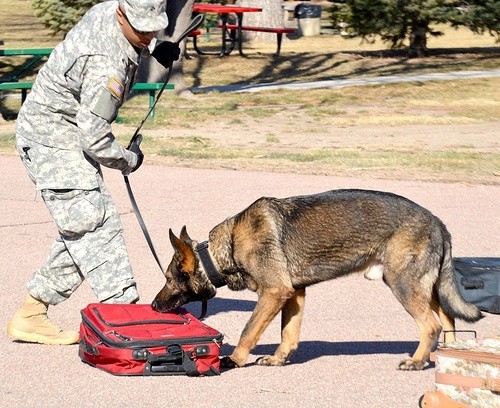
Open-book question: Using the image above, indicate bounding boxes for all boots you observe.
[10,294,80,344]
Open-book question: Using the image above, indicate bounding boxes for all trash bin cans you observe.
[296,3,322,37]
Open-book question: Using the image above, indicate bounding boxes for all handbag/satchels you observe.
[79,302,223,377]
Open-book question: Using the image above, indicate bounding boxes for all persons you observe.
[7,0,180,345]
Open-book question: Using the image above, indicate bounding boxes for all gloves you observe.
[126,134,143,173]
[151,41,180,67]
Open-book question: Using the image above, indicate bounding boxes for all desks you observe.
[0,48,53,82]
[192,4,262,58]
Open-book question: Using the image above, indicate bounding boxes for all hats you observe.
[120,0,169,32]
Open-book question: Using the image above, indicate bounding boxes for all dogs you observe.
[151,189,486,371]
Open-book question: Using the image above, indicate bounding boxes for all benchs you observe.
[188,31,205,55]
[217,25,296,57]
[0,83,175,120]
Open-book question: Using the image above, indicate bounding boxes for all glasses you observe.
[119,8,158,34]
